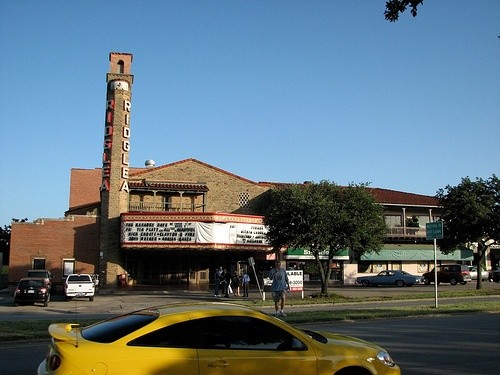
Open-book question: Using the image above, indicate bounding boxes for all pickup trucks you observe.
[62,273,95,302]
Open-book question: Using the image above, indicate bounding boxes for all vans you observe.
[24,269,57,295]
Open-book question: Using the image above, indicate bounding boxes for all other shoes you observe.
[275,312,281,318]
[281,312,287,317]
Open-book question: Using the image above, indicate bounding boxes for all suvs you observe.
[422,263,471,286]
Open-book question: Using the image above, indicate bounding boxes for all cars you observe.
[356,270,426,287]
[486,267,500,283]
[468,265,490,281]
[13,277,51,307]
[37,299,404,375]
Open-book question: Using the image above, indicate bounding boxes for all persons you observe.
[268,260,290,318]
[213,266,241,298]
[241,270,250,297]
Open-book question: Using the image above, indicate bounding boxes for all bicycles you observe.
[226,274,249,298]
